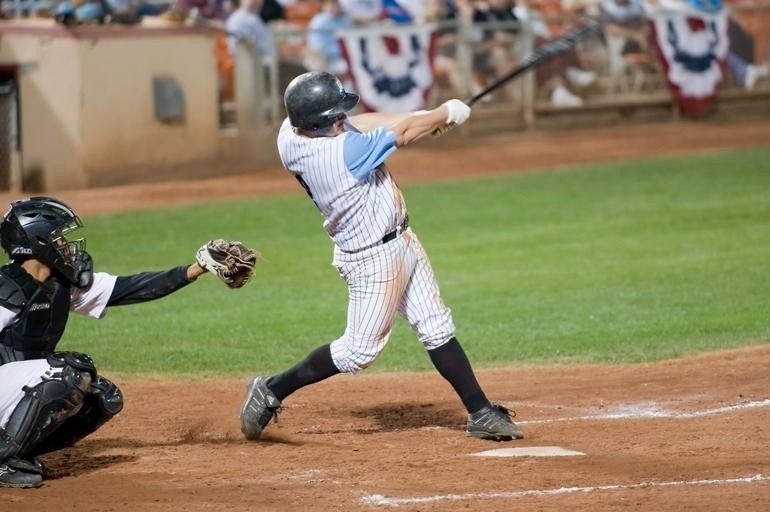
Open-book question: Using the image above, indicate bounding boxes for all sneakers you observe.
[464,400,524,440]
[239,374,287,440]
[0,461,43,488]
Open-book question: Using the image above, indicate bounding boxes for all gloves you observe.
[441,99,472,127]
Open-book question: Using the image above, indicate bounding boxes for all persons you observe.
[236,67,526,443]
[0,193,258,491]
[0,0,770,120]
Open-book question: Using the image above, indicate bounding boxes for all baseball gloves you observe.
[195,238,257,288]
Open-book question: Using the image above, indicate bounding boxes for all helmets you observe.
[0,196,93,293]
[284,71,361,130]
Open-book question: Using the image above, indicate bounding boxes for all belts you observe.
[381,212,409,243]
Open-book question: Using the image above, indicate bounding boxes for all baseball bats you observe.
[432,18,599,139]
